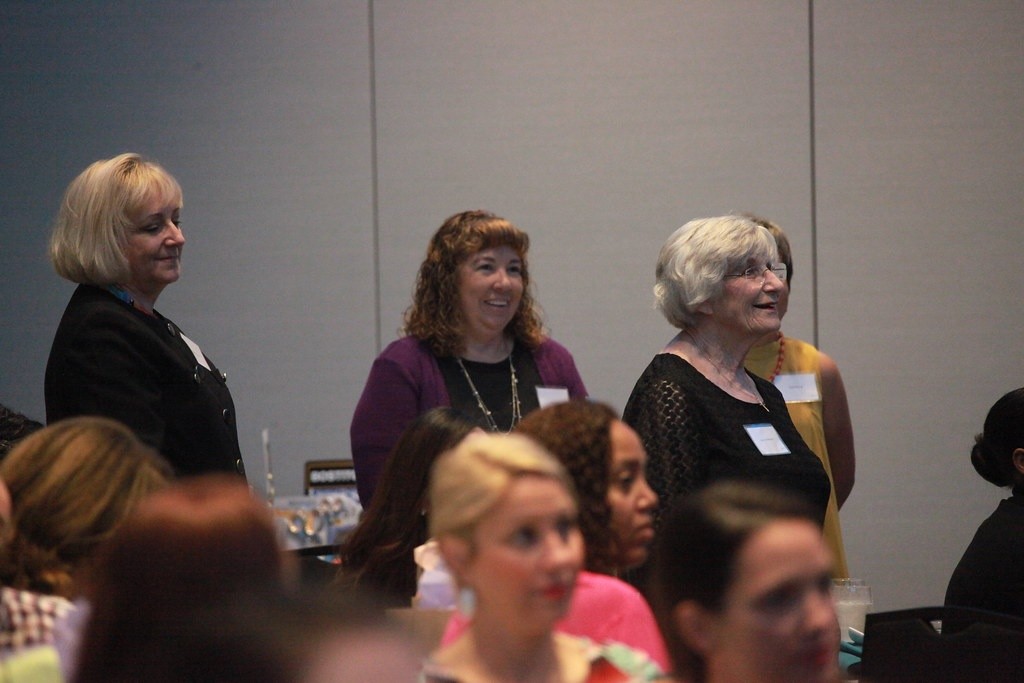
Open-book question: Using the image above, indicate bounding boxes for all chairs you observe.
[859,606,1024,683]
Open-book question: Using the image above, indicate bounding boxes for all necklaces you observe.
[703,351,770,414]
[766,331,785,383]
[455,338,522,436]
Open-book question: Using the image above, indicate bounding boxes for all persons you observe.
[0,416,190,682]
[640,483,840,683]
[418,426,677,683]
[744,216,856,587]
[66,473,315,683]
[337,406,490,627]
[620,214,832,683]
[351,211,588,514]
[42,152,253,491]
[511,401,659,575]
[940,387,1024,683]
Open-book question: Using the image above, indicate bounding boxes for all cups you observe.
[830,577,873,642]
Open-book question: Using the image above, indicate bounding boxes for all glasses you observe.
[724,263,788,281]
